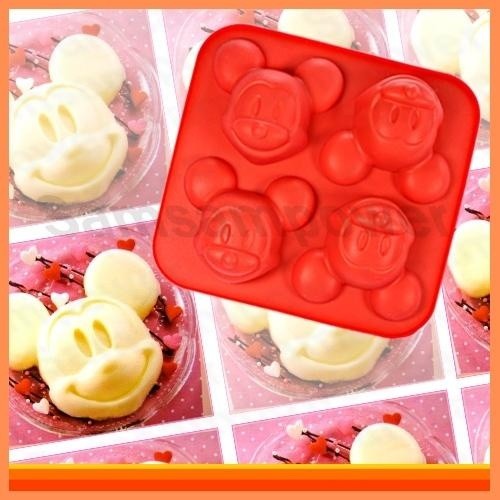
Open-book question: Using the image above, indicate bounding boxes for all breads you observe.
[9,248,165,421]
[350,423,426,464]
[219,297,392,383]
[411,9,490,123]
[447,220,491,297]
[9,34,130,204]
[182,9,355,91]
